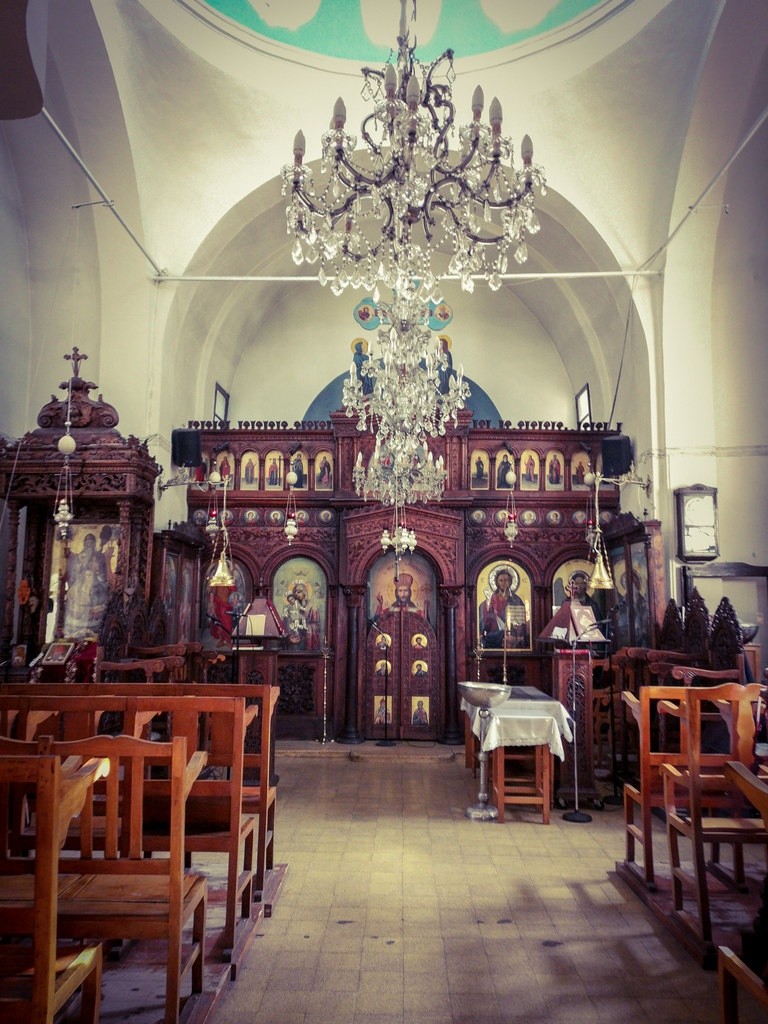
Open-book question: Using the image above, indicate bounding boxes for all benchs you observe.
[620,682,768,1024]
[0,682,280,1024]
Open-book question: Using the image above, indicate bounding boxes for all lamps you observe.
[588,460,652,588]
[53,202,79,541]
[280,0,547,562]
[157,462,238,588]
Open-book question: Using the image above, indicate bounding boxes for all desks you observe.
[742,642,761,683]
[459,686,574,825]
